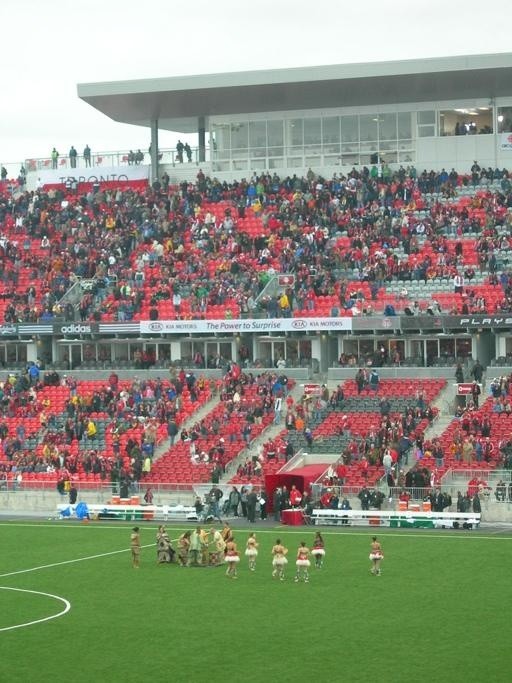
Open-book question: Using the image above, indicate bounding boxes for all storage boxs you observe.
[388,499,434,528]
[108,494,154,519]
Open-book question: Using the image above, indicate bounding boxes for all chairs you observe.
[1,172,511,492]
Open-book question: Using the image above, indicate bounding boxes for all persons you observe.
[130,527,142,569]
[176,140,192,163]
[0,340,512,528]
[311,531,325,569]
[84,145,92,167]
[68,146,76,167]
[156,522,240,578]
[244,534,259,572]
[271,539,287,581]
[295,541,310,582]
[370,153,411,163]
[52,148,59,169]
[456,122,512,135]
[368,536,383,575]
[128,149,144,165]
[1,166,511,324]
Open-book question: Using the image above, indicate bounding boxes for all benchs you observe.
[310,508,483,528]
[54,503,198,519]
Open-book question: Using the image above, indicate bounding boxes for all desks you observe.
[281,508,304,526]
